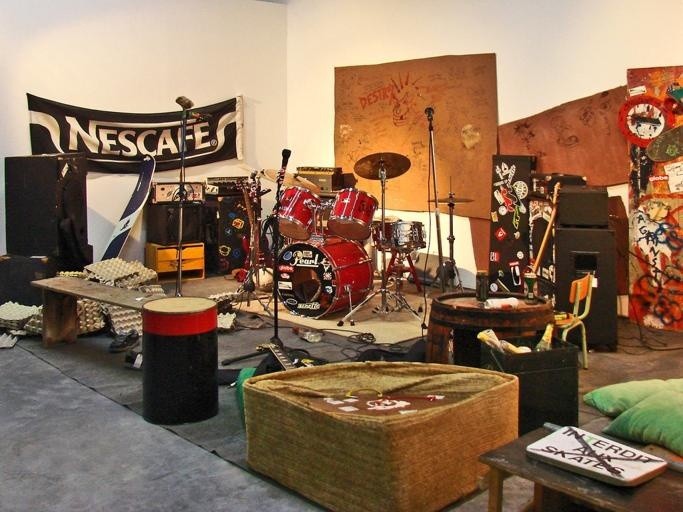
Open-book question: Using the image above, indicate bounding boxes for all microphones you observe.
[425,107,434,130]
[176,95,192,109]
[278,149,291,185]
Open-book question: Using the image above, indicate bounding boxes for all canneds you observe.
[476,270,488,302]
[522,273,538,304]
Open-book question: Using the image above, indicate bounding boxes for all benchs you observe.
[30,277,166,348]
[478,424,683,512]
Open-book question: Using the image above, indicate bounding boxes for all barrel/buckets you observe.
[425,291,557,368]
[141,297,218,425]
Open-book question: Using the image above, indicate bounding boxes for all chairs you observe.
[553,272,598,368]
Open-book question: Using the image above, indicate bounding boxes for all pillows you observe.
[582,375,682,417]
[602,390,683,456]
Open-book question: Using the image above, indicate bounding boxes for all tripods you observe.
[337,172,428,331]
[424,120,465,298]
[222,179,327,373]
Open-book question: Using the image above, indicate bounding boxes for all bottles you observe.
[293,324,320,343]
[484,296,520,312]
[534,323,554,353]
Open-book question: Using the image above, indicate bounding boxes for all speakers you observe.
[558,185,609,227]
[0,245,93,307]
[5,153,87,256]
[146,204,203,245]
[556,228,618,346]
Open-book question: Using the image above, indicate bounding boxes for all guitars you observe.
[521,182,560,294]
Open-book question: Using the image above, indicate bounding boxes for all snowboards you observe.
[100,153,156,261]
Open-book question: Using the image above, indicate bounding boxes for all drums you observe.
[372,218,427,251]
[275,234,374,318]
[328,187,379,241]
[275,186,321,240]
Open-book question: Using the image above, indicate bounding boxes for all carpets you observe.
[231,282,434,347]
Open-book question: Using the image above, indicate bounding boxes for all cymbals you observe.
[265,168,319,192]
[427,197,474,203]
[354,153,411,180]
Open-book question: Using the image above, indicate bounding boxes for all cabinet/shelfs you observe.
[145,243,205,281]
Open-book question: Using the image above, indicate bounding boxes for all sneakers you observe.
[107,330,140,353]
[124,351,143,369]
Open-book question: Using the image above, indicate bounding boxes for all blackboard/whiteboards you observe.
[526,425,668,487]
